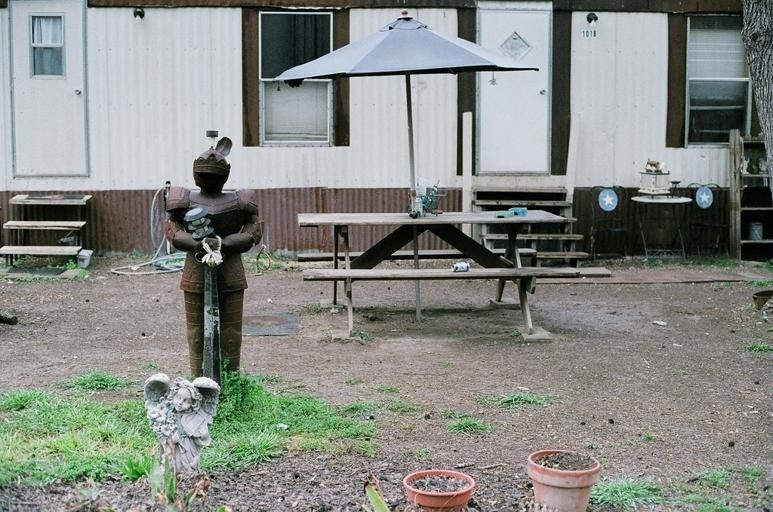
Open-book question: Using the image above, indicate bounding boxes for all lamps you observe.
[586,13,598,27]
[133,8,144,23]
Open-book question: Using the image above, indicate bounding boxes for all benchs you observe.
[297,248,538,262]
[302,266,577,281]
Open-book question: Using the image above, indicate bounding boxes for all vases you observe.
[403,470,475,512]
[526,449,600,512]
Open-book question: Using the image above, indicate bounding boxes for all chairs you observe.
[586,186,630,263]
[684,182,730,257]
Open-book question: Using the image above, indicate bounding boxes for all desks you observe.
[631,195,693,260]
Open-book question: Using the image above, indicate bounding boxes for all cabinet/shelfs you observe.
[729,129,773,262]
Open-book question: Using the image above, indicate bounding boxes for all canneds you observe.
[451,262,470,272]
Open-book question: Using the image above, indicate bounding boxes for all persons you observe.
[144,373,221,478]
[163,136,262,379]
[273,9,539,212]
